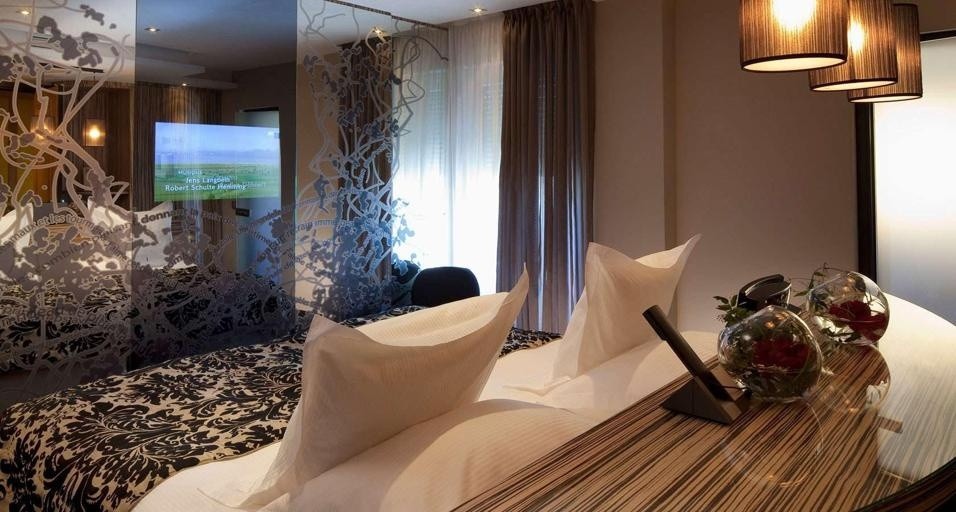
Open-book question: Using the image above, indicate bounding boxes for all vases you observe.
[716,306,824,402]
[806,272,890,347]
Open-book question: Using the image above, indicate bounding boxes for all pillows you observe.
[259,398,596,511]
[504,233,702,394]
[540,331,720,422]
[198,263,529,506]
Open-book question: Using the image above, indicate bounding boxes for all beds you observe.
[1,306,720,512]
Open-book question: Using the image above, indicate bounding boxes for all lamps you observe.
[737,0,847,72]
[809,0,898,92]
[847,3,923,102]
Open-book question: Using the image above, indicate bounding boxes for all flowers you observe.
[794,263,886,345]
[715,294,818,397]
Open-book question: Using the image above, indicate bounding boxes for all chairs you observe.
[411,268,480,307]
